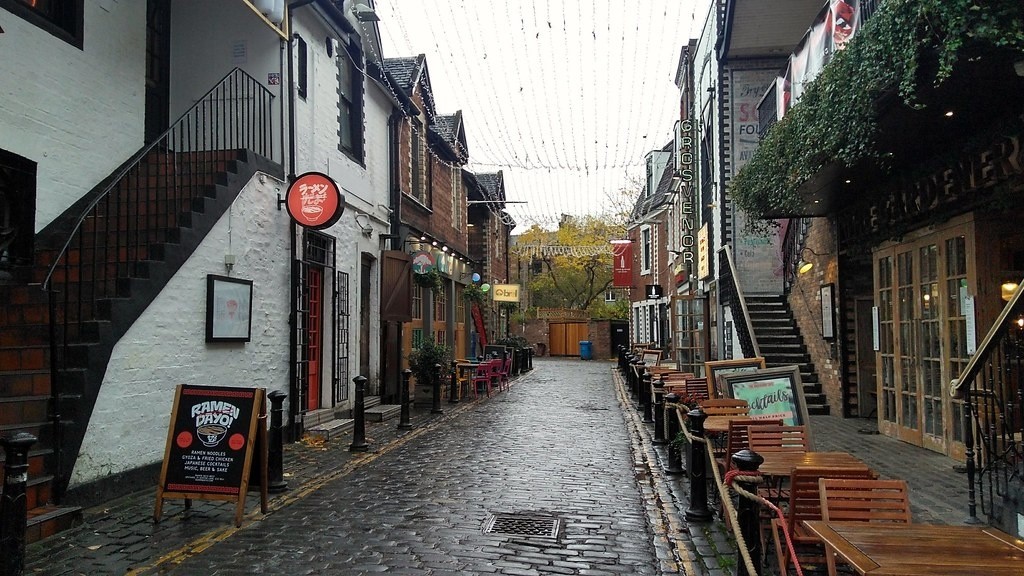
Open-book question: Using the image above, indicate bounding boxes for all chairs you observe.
[644,365,912,576]
[442,358,512,400]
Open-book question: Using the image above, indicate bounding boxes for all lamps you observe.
[354,211,372,232]
[797,247,828,273]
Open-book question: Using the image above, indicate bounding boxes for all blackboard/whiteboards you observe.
[155,383,273,505]
[482,344,514,377]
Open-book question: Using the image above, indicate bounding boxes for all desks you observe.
[704,416,753,502]
[800,517,1024,576]
[662,381,686,394]
[752,451,870,568]
[456,364,480,398]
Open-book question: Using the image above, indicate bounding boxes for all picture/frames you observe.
[703,357,766,417]
[717,365,815,453]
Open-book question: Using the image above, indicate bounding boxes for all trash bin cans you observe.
[579,340,593,361]
